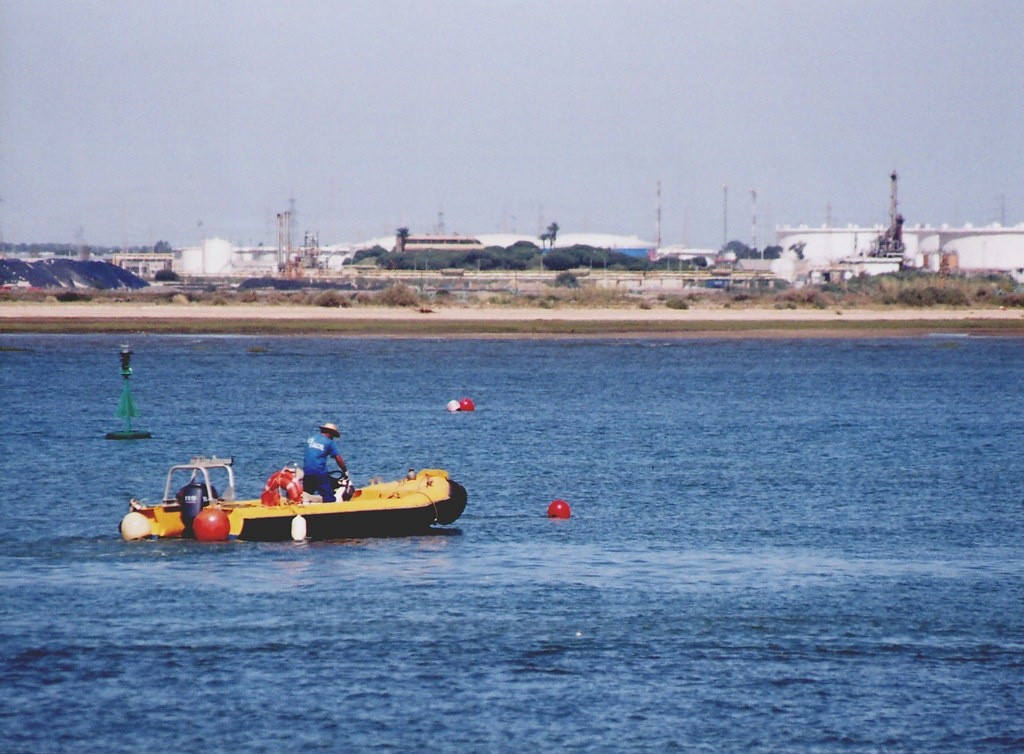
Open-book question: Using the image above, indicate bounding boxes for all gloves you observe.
[339,471,350,481]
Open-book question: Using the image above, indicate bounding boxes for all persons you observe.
[303,422,350,503]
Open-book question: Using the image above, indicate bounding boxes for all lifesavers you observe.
[257,467,305,510]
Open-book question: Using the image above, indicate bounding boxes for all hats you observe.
[319,423,340,437]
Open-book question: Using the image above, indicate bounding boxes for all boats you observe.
[118,454,468,543]
